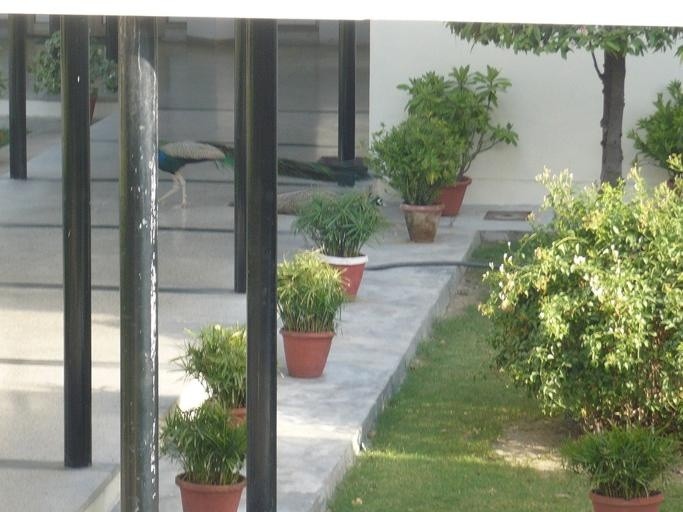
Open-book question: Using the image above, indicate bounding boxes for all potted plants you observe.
[397,64,519,217]
[274,247,351,378]
[175,324,248,432]
[560,425,678,512]
[370,116,465,243]
[27,31,119,124]
[626,80,683,189]
[292,188,386,300]
[158,401,248,512]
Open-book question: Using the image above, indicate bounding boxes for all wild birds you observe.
[158,141,358,209]
